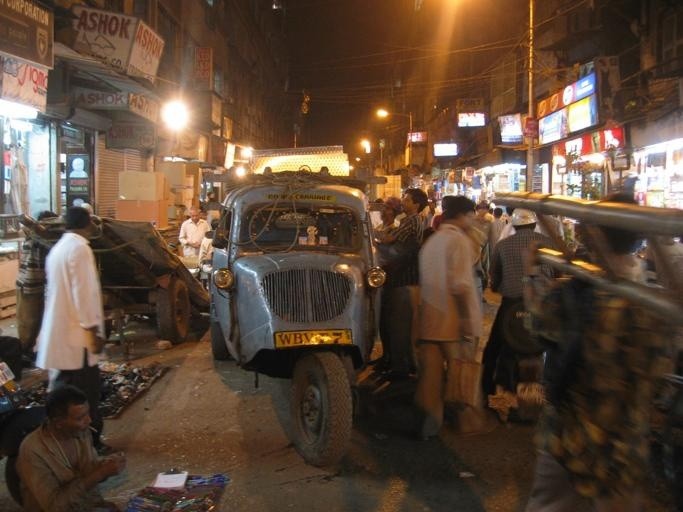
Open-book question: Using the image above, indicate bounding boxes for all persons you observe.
[515,194,674,512]
[178,192,222,274]
[35,206,112,453]
[15,384,127,512]
[367,188,563,438]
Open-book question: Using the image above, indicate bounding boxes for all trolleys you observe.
[18,213,209,343]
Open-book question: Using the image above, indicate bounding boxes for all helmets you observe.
[511,208,538,226]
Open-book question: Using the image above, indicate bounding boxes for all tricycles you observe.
[203,182,383,465]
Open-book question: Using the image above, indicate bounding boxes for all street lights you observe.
[376,109,413,166]
[360,139,383,171]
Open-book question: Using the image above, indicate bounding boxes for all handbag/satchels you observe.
[376,234,420,274]
